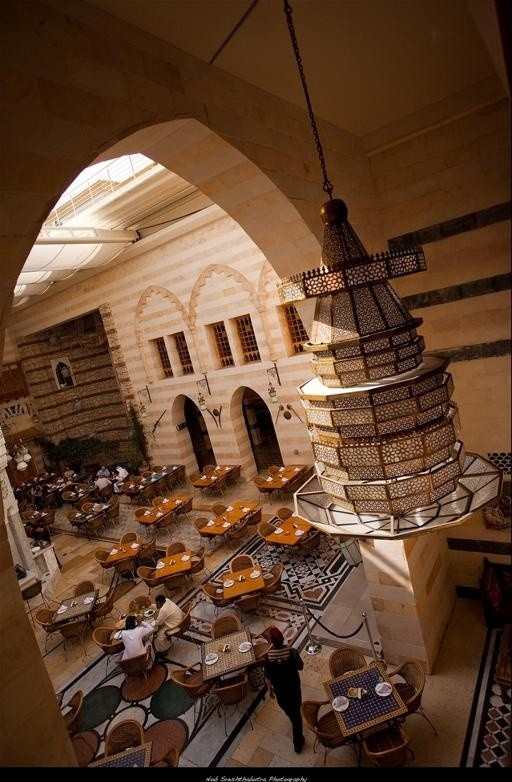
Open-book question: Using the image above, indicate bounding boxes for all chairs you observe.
[13,465,438,767]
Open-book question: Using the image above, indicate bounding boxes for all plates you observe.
[33,511,48,517]
[155,562,165,569]
[250,571,260,578]
[114,630,122,640]
[332,696,350,713]
[205,652,218,666]
[142,497,182,517]
[83,597,94,605]
[274,528,284,534]
[129,466,178,489]
[144,609,155,617]
[374,682,393,697]
[206,504,251,527]
[16,472,57,491]
[180,555,190,561]
[294,529,305,535]
[130,543,139,548]
[109,548,118,555]
[239,641,252,653]
[200,465,231,481]
[57,608,67,614]
[267,466,301,482]
[223,580,235,587]
[74,502,109,518]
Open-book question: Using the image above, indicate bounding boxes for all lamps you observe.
[0,410,32,472]
[196,375,211,410]
[267,363,281,403]
[137,386,153,418]
[277,0,505,538]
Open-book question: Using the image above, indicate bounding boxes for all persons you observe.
[96,464,110,477]
[95,475,113,502]
[65,467,75,478]
[146,594,186,656]
[114,466,130,494]
[121,615,156,672]
[262,626,305,753]
[31,481,44,508]
